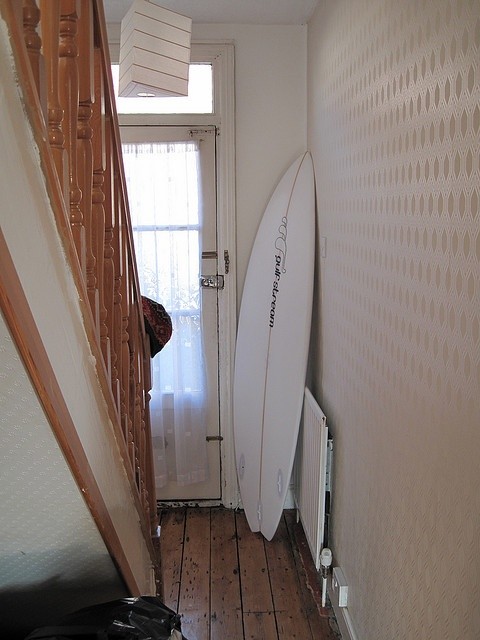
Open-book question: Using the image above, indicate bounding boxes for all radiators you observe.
[289,383,328,574]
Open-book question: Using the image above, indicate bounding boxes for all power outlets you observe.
[328,567,349,609]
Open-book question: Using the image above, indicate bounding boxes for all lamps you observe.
[114,1,195,100]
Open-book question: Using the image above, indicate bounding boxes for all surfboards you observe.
[230,147,318,546]
